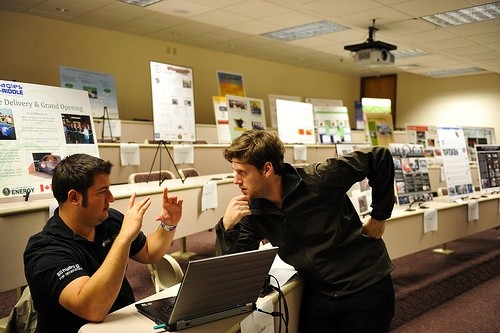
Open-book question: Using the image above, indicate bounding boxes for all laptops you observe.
[135,246,279,332]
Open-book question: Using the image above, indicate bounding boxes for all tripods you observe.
[146,140,184,186]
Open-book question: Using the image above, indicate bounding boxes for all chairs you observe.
[147,253,184,293]
[177,168,200,178]
[129,170,176,184]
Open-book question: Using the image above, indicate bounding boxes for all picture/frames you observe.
[216,70,246,97]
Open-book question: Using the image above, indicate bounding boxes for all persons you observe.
[215,130,395,332]
[23,153,183,333]
[64,120,94,144]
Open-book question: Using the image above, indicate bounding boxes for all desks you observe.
[0,120,500,333]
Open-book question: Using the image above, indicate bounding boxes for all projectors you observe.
[353,48,394,67]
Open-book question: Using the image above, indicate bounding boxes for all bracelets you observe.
[161,220,178,231]
